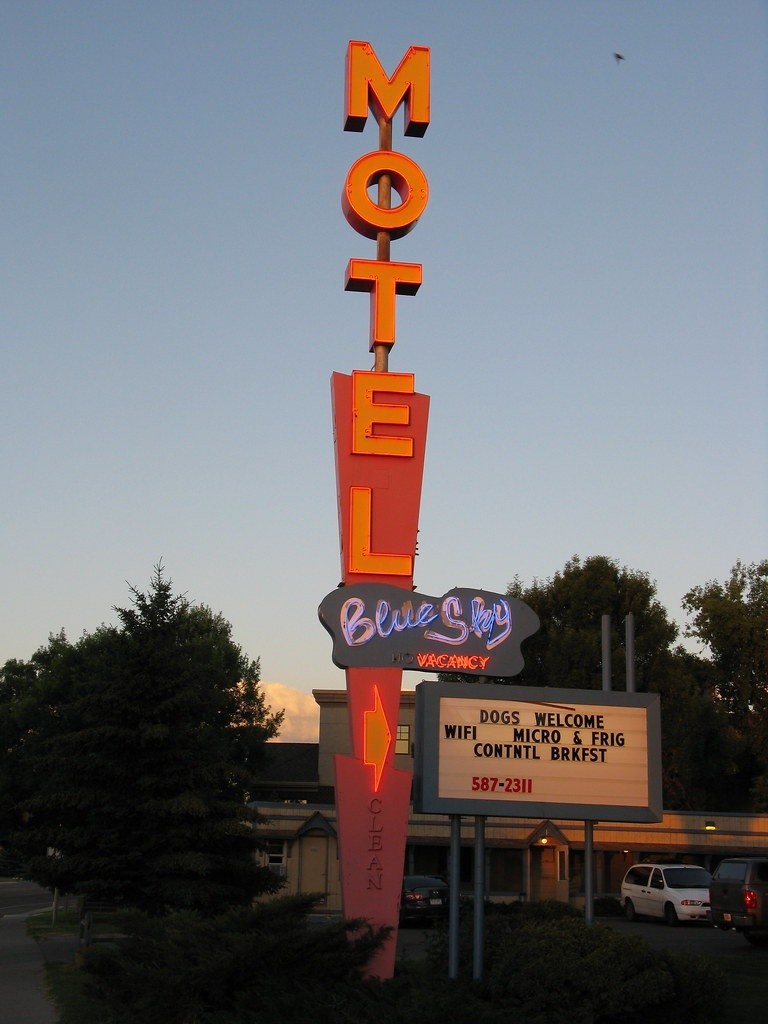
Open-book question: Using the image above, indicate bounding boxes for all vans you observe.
[708,856,768,948]
[620,863,713,928]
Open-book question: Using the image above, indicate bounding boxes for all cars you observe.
[397,874,449,929]
